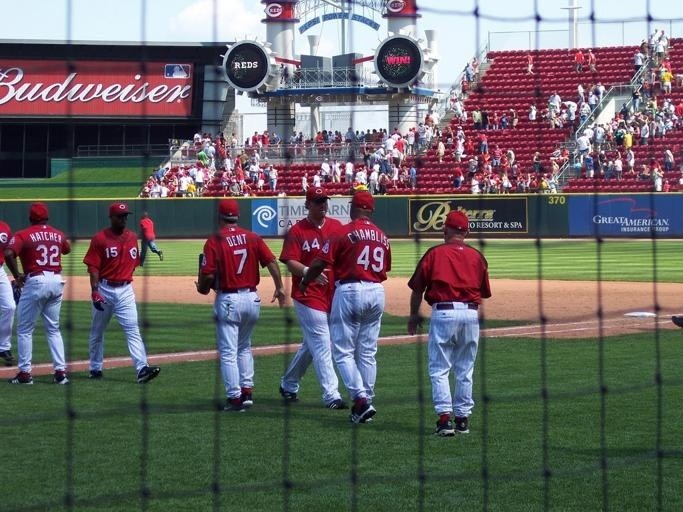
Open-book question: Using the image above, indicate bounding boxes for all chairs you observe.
[139,134,393,197]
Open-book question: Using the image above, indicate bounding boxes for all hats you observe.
[347,184,374,210]
[218,199,240,217]
[444,210,469,231]
[30,202,50,221]
[306,187,330,202]
[109,202,134,216]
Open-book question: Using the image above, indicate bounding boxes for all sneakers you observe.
[432,420,456,437]
[137,366,160,383]
[47,371,70,385]
[224,394,252,412]
[452,417,470,434]
[9,371,33,385]
[326,399,349,408]
[348,399,377,426]
[279,386,301,406]
[0,349,15,362]
[89,370,103,380]
[157,250,163,261]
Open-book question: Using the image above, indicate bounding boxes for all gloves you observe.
[92,290,108,312]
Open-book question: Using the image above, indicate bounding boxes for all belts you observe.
[437,303,477,309]
[100,278,130,288]
[335,278,370,287]
[220,286,259,293]
[29,269,60,276]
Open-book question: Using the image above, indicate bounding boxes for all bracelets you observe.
[302,267,309,277]
[301,277,308,286]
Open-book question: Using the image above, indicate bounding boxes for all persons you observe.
[194,200,286,412]
[139,212,163,266]
[407,211,491,436]
[279,188,351,409]
[432,29,683,194]
[4,203,70,385]
[279,62,304,83]
[299,193,391,423]
[83,205,160,384]
[0,221,17,362]
[140,108,440,197]
